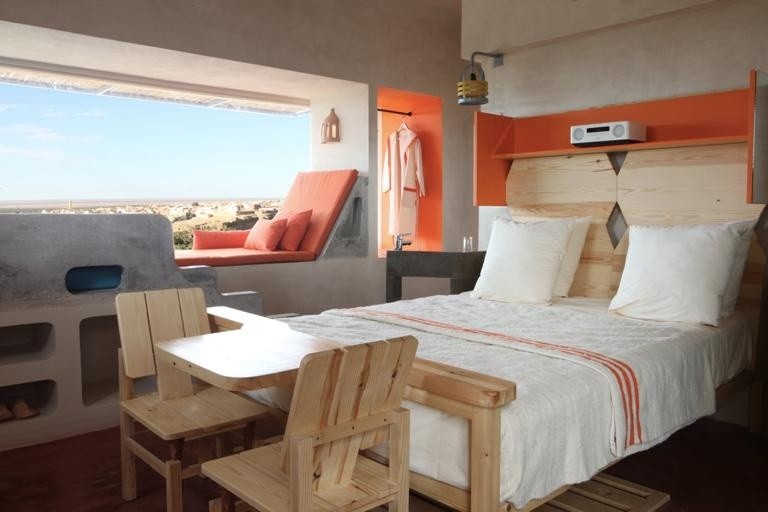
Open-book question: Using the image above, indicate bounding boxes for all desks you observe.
[153,301,347,394]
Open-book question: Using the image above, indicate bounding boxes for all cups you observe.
[463,236,473,252]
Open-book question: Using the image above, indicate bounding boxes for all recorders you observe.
[570,120,647,146]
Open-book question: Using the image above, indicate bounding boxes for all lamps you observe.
[319,107,340,144]
[454,48,503,106]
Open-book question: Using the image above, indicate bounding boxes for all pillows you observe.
[720,215,758,320]
[244,217,291,251]
[496,215,592,299]
[271,208,318,252]
[469,214,577,302]
[610,218,747,329]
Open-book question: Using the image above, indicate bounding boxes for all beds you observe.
[235,150,764,511]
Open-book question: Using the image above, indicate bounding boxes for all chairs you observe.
[202,334,424,512]
[110,286,268,512]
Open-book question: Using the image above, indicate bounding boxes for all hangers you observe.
[392,112,412,141]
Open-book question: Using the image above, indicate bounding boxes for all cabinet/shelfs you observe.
[0,294,162,452]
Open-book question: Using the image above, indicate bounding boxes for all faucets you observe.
[397,232,412,251]
